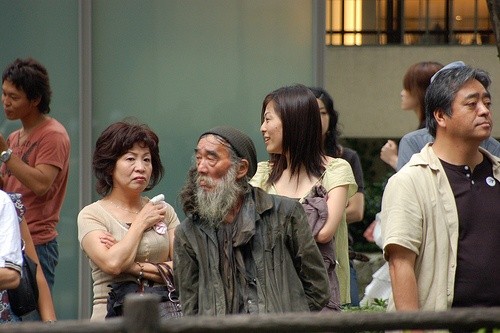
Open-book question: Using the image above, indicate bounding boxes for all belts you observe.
[348,252,369,262]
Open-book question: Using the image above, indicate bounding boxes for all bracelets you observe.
[136,261,144,278]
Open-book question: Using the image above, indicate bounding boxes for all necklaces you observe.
[107,197,144,214]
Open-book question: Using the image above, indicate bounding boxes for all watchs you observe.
[0,148,12,162]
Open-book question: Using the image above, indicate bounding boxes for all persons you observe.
[171,127,333,314]
[380,66,500,333]
[7,192,59,325]
[358,61,500,312]
[245,84,358,313]
[307,86,364,307]
[77,117,180,321]
[0,188,24,323]
[0,56,71,322]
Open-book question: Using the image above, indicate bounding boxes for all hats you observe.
[198,128,258,181]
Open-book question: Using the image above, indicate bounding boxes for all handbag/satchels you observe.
[7,239,38,317]
[153,262,183,319]
